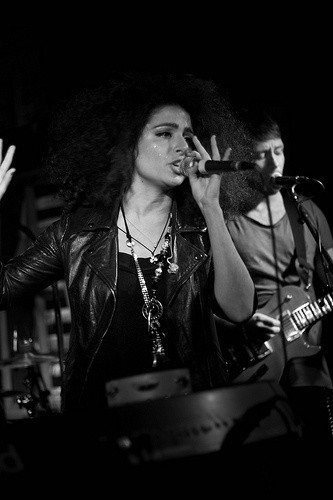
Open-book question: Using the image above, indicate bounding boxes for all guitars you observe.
[219,282,333,385]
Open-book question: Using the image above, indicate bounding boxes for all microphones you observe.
[180,156,256,177]
[269,172,309,188]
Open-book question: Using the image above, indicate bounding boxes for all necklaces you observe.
[115,198,175,368]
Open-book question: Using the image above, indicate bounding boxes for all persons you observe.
[0,73,259,417]
[226,116,333,388]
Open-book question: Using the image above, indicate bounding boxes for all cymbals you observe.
[1,336,59,371]
